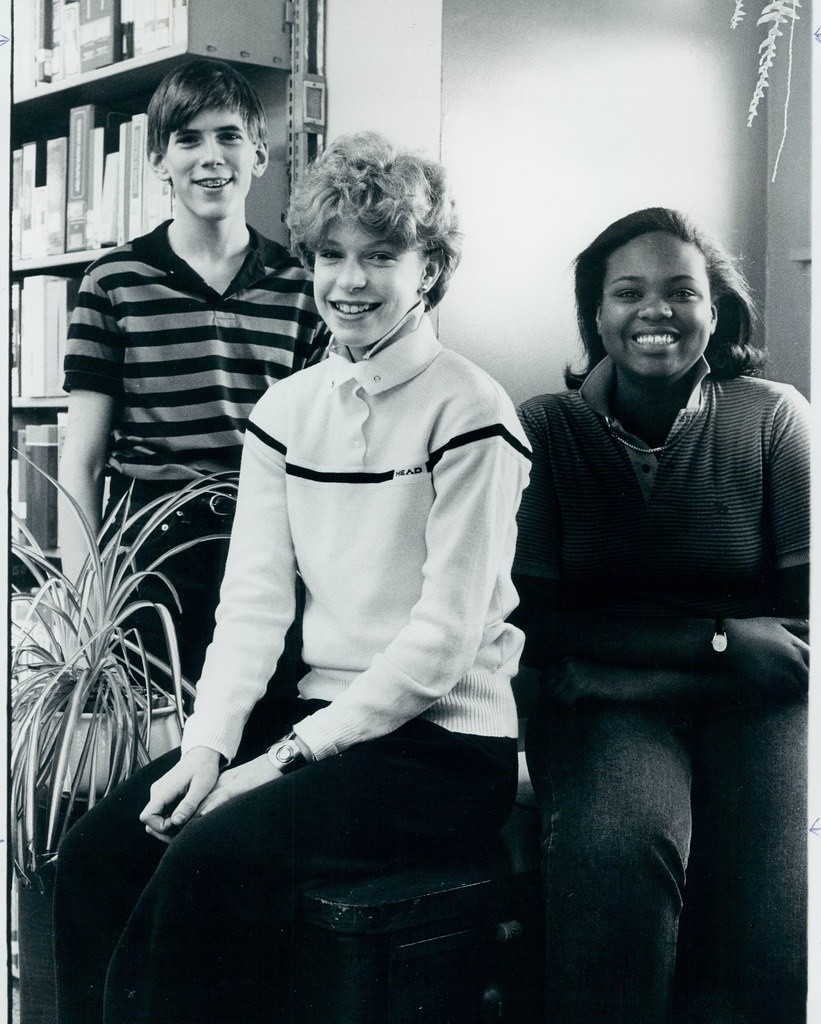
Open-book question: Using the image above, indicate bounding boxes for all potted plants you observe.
[9,447,241,888]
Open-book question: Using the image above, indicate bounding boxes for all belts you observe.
[109,474,238,517]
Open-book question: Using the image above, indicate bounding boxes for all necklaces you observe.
[606,388,704,452]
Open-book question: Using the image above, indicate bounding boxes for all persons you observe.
[59,59,333,710]
[52,129,525,1024]
[519,208,810,1024]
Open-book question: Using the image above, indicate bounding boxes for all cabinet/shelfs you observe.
[0,0,328,573]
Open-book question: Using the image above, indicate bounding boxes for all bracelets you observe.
[266,732,308,776]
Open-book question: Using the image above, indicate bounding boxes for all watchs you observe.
[713,617,727,666]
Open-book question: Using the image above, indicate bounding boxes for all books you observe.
[12,0,187,554]
